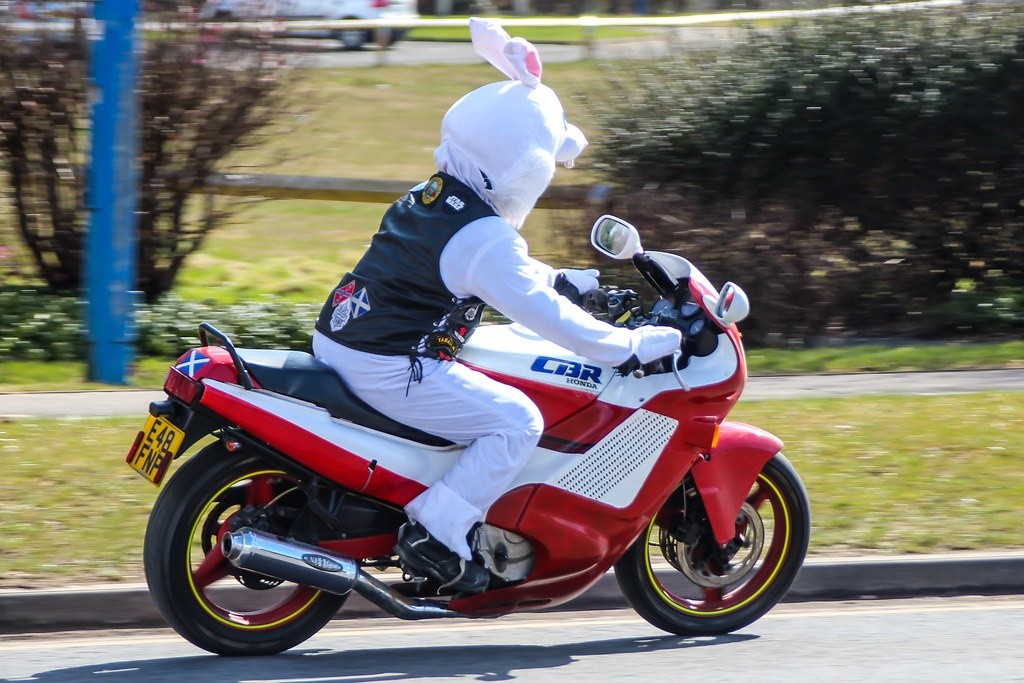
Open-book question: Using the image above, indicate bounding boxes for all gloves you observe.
[557,268,600,295]
[633,325,681,364]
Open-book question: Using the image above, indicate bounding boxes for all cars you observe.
[198,0,423,49]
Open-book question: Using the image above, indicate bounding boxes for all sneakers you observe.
[392,520,491,592]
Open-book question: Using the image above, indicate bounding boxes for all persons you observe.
[313,17,682,597]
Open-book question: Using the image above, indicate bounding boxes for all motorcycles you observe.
[126,214,811,658]
